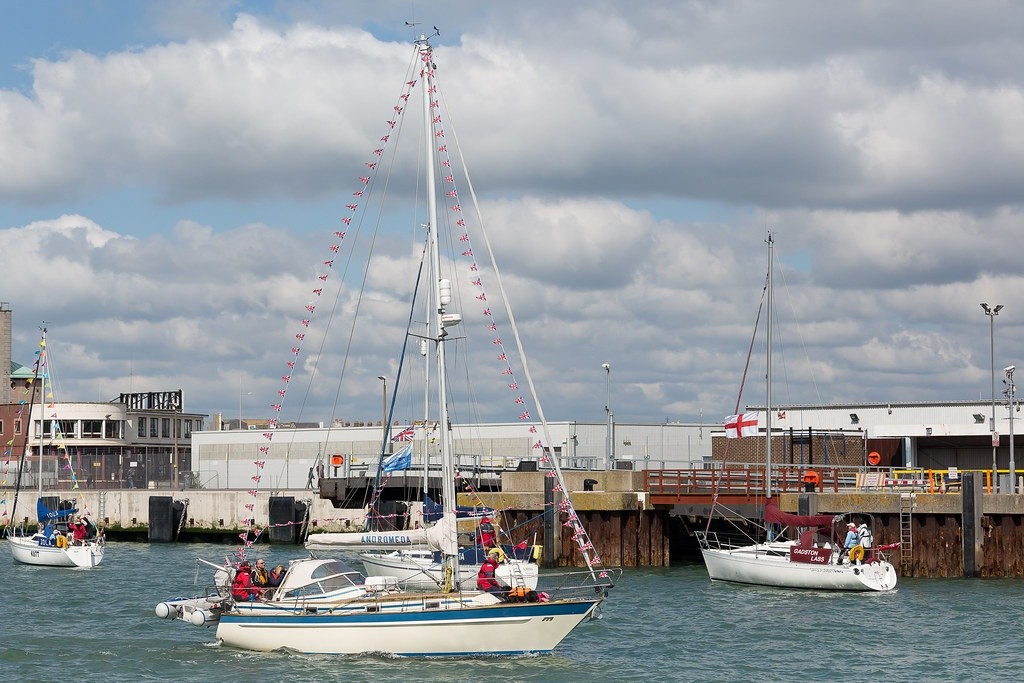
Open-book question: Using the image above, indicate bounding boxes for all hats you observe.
[847,523,855,527]
[861,523,867,528]
[53,530,58,534]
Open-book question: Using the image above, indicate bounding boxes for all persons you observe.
[836,523,860,566]
[316,460,324,489]
[497,585,550,603]
[69,518,86,546]
[469,517,496,548]
[477,553,507,601]
[231,561,265,602]
[308,467,315,489]
[833,515,848,551]
[251,559,274,600]
[415,520,418,529]
[856,523,872,565]
[48,528,62,547]
[269,563,294,601]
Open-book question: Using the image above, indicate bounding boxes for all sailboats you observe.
[693,228,902,593]
[0,321,107,567]
[154,17,623,667]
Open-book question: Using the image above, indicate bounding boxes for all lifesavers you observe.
[56,535,67,548]
[486,548,505,562]
[850,545,863,561]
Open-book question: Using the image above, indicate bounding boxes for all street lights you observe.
[1001,366,1020,495]
[601,362,612,470]
[376,375,387,444]
[980,302,1004,496]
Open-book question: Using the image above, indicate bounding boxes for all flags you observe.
[777,411,785,420]
[379,440,411,471]
[513,538,528,552]
[725,411,758,438]
[390,425,414,442]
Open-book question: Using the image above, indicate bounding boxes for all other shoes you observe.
[837,563,842,565]
